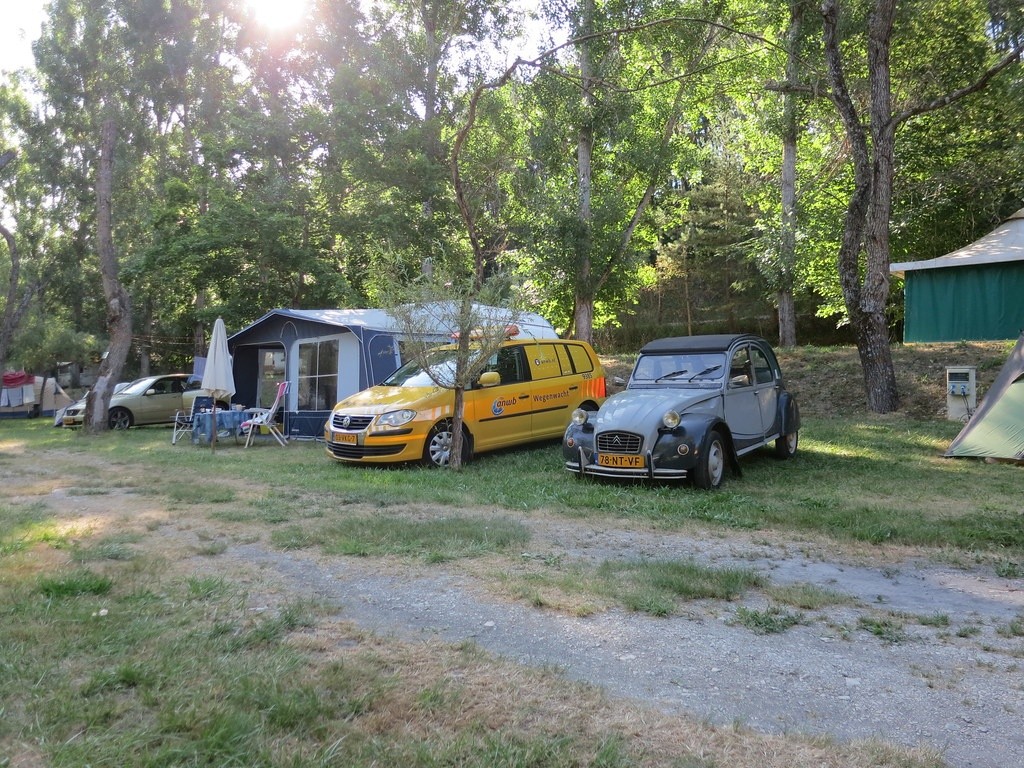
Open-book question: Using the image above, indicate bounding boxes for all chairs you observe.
[170,396,214,446]
[239,381,290,448]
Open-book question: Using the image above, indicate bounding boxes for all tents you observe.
[887,206,1024,343]
[222,296,559,441]
[944,329,1024,461]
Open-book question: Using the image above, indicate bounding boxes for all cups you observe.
[201,407,206,412]
[205,409,211,413]
[236,405,242,412]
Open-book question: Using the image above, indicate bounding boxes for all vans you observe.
[321,324,608,465]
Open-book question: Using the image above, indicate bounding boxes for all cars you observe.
[64,372,205,432]
[561,333,801,491]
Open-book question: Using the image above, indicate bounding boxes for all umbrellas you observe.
[200,315,236,455]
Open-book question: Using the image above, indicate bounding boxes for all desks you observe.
[191,408,252,448]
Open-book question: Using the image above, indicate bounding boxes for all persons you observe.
[212,394,233,438]
